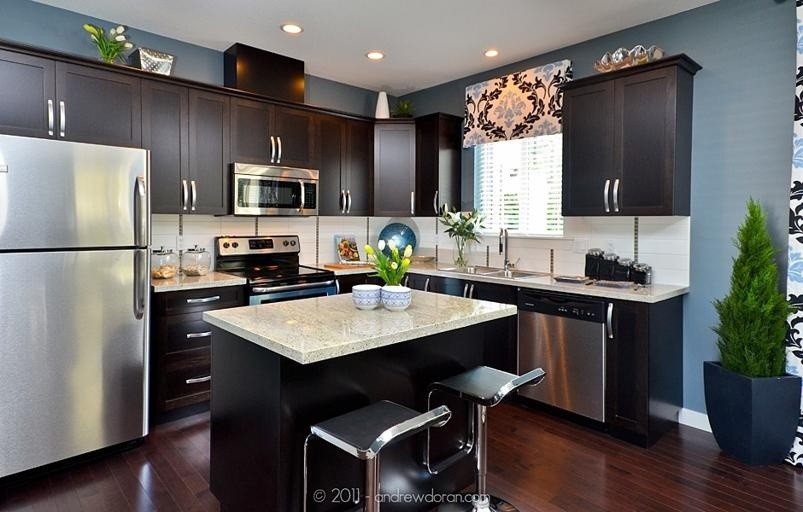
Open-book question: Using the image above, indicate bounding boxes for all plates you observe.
[406,254,434,264]
[438,267,456,271]
[379,223,416,261]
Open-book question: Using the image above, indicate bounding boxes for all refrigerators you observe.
[0,133,152,478]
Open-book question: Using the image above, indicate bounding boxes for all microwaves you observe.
[229,163,320,217]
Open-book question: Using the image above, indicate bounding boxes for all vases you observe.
[375,90,390,119]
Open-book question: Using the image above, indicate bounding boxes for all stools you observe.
[424,364,546,510]
[301,398,452,510]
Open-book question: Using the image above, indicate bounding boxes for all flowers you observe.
[85,17,135,66]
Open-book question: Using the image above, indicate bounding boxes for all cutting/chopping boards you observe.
[323,262,376,270]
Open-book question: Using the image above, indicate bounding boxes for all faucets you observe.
[498,227,515,269]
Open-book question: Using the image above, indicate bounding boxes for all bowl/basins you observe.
[350,283,413,313]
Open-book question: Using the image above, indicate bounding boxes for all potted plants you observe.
[390,96,416,117]
[705,196,802,459]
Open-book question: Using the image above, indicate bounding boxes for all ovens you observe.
[247,283,338,306]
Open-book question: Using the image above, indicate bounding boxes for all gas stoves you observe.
[216,264,337,293]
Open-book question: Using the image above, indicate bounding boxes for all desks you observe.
[202,284,520,512]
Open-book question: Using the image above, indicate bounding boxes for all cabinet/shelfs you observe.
[1,37,140,148]
[145,71,226,209]
[314,112,374,218]
[606,296,688,437]
[559,52,702,216]
[228,95,316,166]
[374,118,441,217]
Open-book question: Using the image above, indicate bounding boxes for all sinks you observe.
[438,264,502,275]
[477,268,551,282]
